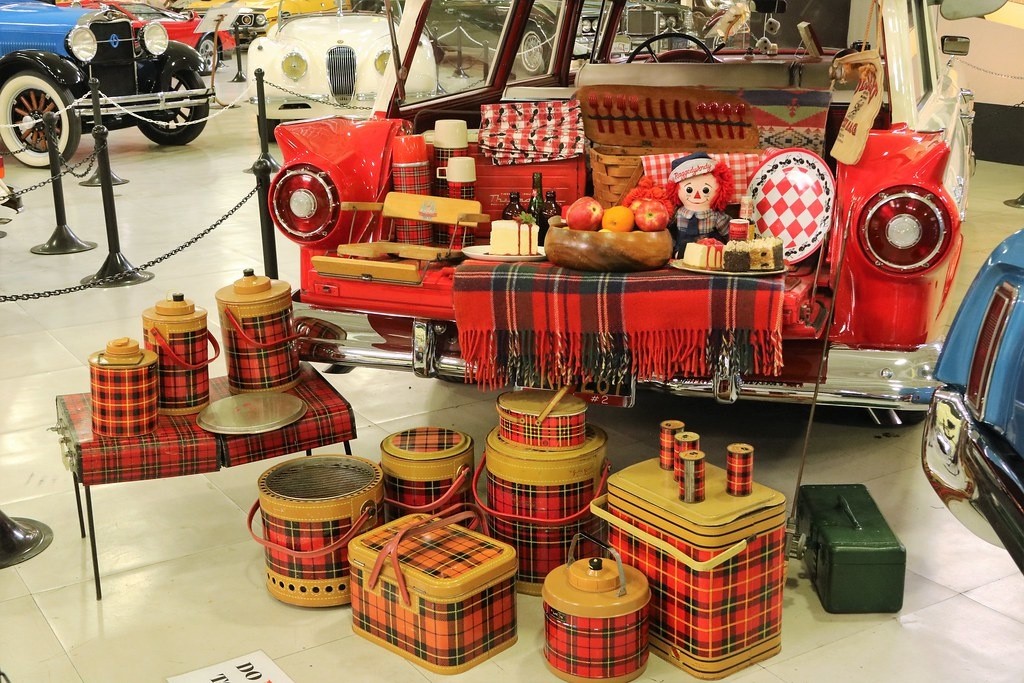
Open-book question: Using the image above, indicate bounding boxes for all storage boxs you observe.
[588,147,763,210]
[590,457,785,679]
[796,484,907,614]
[420,128,586,238]
[247,384,652,683]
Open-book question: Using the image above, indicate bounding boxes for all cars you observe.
[268,0,1008,425]
[163,0,351,50]
[0,0,218,169]
[925,1,1022,108]
[55,0,236,76]
[921,230,1023,576]
[351,0,706,77]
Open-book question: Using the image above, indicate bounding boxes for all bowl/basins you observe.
[544,223,673,272]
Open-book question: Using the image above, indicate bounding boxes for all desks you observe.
[45,361,359,602]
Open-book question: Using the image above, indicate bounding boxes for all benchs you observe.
[501,61,890,111]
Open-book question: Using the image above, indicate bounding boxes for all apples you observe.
[560,195,669,234]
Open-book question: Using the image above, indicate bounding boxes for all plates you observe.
[461,245,546,260]
[672,261,788,275]
[746,151,836,263]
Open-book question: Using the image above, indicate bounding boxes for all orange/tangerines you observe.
[602,205,635,233]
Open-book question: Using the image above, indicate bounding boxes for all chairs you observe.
[308,191,491,287]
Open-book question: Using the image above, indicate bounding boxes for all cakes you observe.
[722,236,783,270]
[682,237,728,271]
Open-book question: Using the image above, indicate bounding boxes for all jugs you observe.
[432,120,469,246]
[436,157,477,247]
[392,135,432,248]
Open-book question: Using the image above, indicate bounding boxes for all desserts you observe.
[488,211,540,255]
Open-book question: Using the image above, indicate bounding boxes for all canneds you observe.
[678,450,706,504]
[659,419,685,470]
[673,432,700,483]
[726,442,754,496]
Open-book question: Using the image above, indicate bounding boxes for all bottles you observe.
[540,191,561,245]
[502,192,525,220]
[527,172,544,246]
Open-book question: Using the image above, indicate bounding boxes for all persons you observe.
[668,151,735,259]
